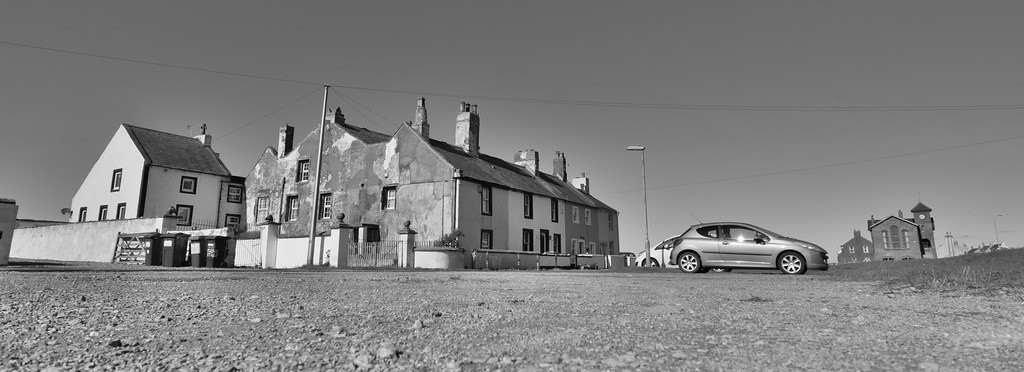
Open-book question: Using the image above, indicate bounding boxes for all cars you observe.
[635,235,733,273]
[669,222,829,275]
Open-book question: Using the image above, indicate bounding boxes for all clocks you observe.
[919,214,925,219]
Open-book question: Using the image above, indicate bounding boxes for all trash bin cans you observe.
[189,235,209,268]
[144,232,162,266]
[204,235,231,268]
[160,232,191,267]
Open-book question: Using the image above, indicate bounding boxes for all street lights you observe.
[625,146,652,269]
